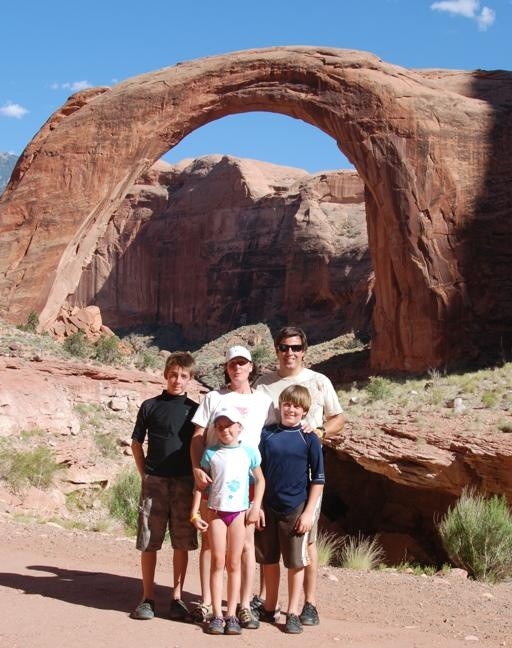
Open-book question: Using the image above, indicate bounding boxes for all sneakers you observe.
[130,598,156,619]
[190,604,213,624]
[236,595,265,613]
[168,599,190,619]
[206,616,226,635]
[238,608,260,629]
[283,614,302,634]
[299,601,319,626]
[251,601,281,622]
[224,614,241,634]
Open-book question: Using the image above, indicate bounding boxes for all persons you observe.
[251,326,345,626]
[259,385,325,634]
[131,353,200,620]
[191,345,277,630]
[189,408,266,636]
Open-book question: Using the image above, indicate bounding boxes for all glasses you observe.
[277,343,303,353]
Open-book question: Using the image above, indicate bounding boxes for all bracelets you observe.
[190,513,202,523]
[315,426,328,439]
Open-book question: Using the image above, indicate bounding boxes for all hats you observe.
[214,406,245,429]
[225,346,252,363]
